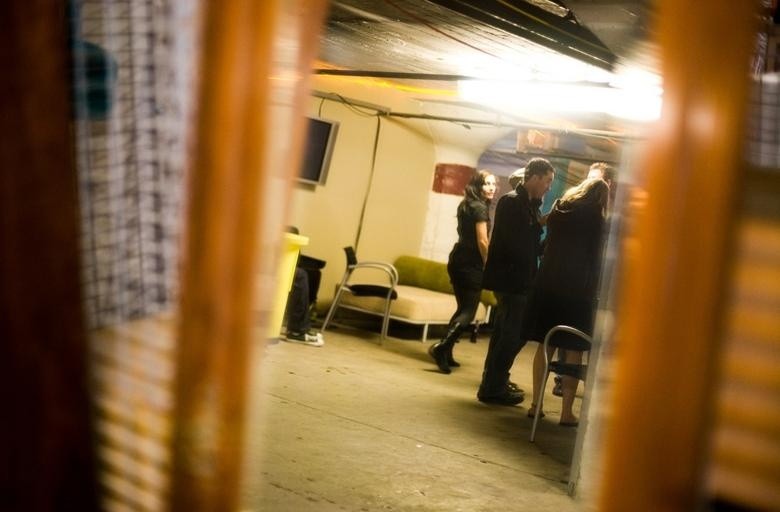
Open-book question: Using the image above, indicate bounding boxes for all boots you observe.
[429,319,464,375]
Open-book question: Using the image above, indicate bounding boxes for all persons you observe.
[477,157,618,425]
[284,227,324,346]
[429,170,499,373]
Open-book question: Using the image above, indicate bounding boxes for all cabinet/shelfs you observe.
[262,232,310,345]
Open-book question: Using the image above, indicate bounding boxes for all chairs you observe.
[529,325,593,444]
[320,247,398,345]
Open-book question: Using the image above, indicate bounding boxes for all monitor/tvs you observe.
[290,115,340,188]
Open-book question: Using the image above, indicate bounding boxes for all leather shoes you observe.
[560,416,580,426]
[528,405,545,418]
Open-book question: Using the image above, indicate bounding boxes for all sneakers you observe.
[287,331,325,347]
[553,377,563,397]
[476,379,526,406]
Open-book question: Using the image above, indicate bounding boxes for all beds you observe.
[336,255,497,343]
